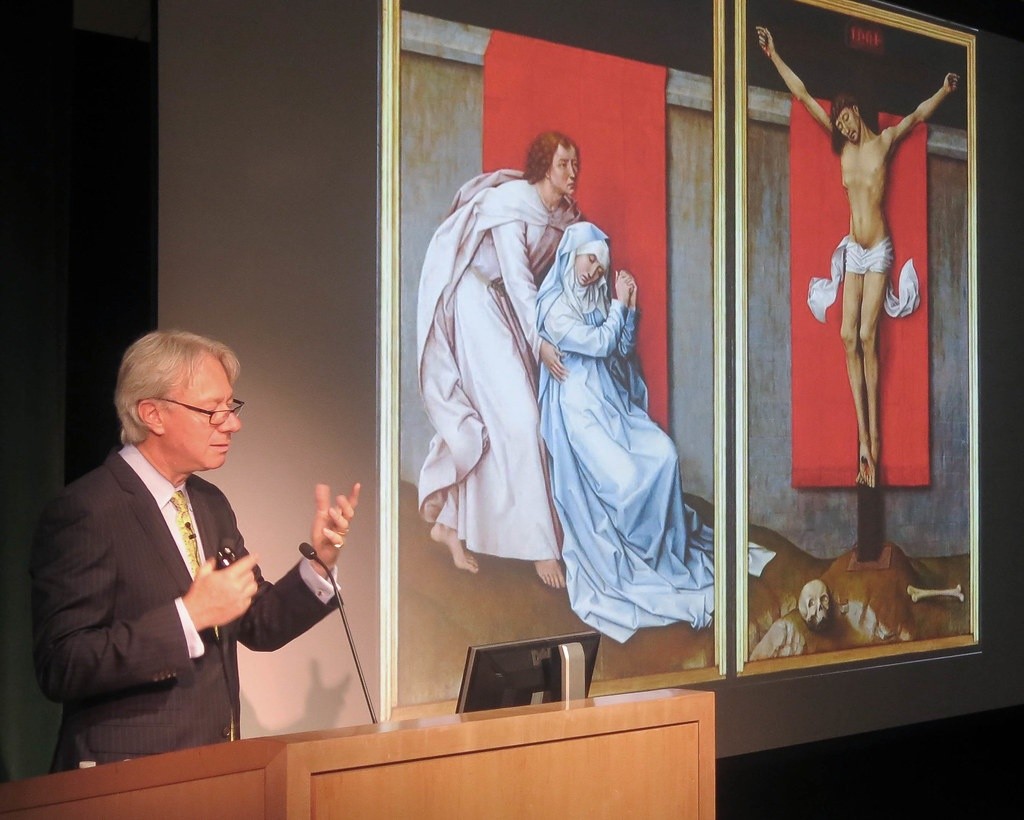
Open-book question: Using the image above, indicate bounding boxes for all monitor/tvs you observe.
[454,627,602,714]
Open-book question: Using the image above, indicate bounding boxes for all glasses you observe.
[137,396,245,426]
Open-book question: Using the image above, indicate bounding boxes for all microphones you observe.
[297,542,378,723]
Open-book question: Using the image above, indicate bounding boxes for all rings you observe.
[336,529,350,535]
[331,543,343,551]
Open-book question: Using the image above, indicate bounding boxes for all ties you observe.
[171,491,219,637]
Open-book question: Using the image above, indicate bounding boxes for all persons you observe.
[29,329,360,775]
